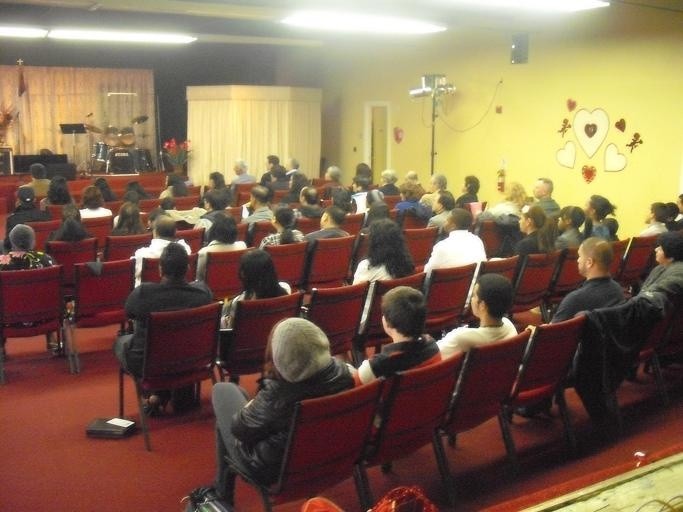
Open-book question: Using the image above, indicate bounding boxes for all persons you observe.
[210,318,357,506]
[0,224,76,361]
[435,274,521,363]
[113,243,213,412]
[4,158,307,328]
[332,283,442,457]
[634,196,683,296]
[510,236,628,420]
[297,164,616,310]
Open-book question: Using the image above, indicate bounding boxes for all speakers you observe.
[47,163,76,180]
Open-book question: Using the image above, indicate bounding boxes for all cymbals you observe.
[85,124,103,136]
[131,116,149,126]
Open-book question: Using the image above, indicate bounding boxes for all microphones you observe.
[87,112,93,117]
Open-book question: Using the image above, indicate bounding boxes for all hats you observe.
[272,317,333,383]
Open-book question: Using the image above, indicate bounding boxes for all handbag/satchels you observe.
[190,486,236,512]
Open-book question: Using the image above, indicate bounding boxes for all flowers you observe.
[159,137,192,164]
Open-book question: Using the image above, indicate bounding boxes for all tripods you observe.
[77,131,93,172]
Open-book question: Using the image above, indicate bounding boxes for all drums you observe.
[94,141,108,162]
[104,126,120,144]
[131,147,153,173]
[121,128,135,145]
[106,147,132,174]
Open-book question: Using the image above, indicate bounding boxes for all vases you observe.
[170,163,185,175]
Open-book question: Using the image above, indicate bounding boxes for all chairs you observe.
[0,176,682,512]
